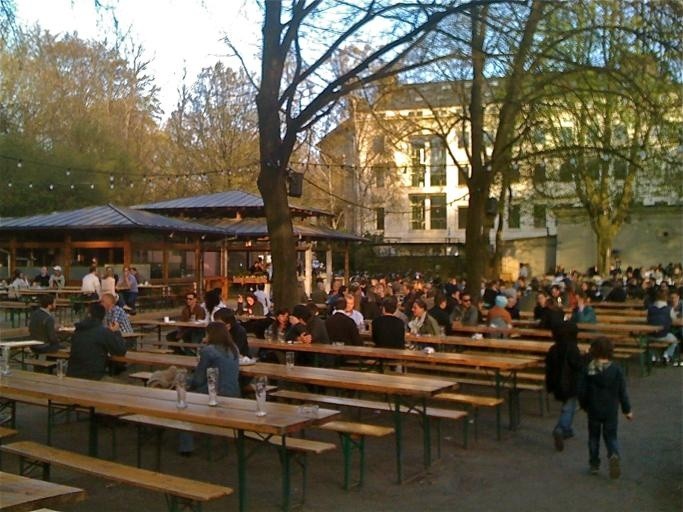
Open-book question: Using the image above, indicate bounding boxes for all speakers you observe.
[289,172,303,198]
[487,198,497,216]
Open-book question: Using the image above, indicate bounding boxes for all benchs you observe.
[0,276,683,512]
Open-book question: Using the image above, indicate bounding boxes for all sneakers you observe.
[591,466,599,473]
[552,426,574,452]
[609,455,621,478]
[652,353,682,367]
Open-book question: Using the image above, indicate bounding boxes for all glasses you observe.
[463,299,472,303]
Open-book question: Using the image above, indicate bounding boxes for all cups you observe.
[191,315,196,322]
[57,359,66,377]
[264,329,273,339]
[1,348,13,376]
[286,352,294,372]
[174,367,267,417]
[164,316,169,323]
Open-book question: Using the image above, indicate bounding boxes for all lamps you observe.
[200,233,207,240]
[245,236,252,247]
[168,230,176,239]
[299,231,302,240]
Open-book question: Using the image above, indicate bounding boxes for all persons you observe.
[2,256,525,398]
[544,320,635,468]
[528,264,682,365]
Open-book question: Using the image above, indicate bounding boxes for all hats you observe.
[496,295,508,308]
